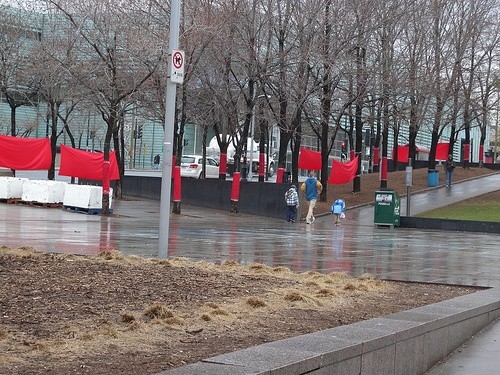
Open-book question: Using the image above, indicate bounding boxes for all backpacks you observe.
[332,199,343,215]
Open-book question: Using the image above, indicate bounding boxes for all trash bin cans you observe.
[428,169,439,187]
[283,172,291,182]
[485,152,498,163]
[374,188,401,227]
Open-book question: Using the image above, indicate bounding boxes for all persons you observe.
[330,198,346,227]
[300,172,322,224]
[154,154,160,170]
[284,185,299,223]
[445,154,456,187]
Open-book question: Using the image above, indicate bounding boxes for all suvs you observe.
[180,155,222,180]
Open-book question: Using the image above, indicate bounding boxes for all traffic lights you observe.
[341,139,349,154]
[143,147,147,156]
[134,128,143,139]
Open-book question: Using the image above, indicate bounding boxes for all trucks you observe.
[201,133,276,177]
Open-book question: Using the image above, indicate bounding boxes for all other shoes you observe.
[289,219,296,223]
[306,217,315,224]
[335,222,341,226]
